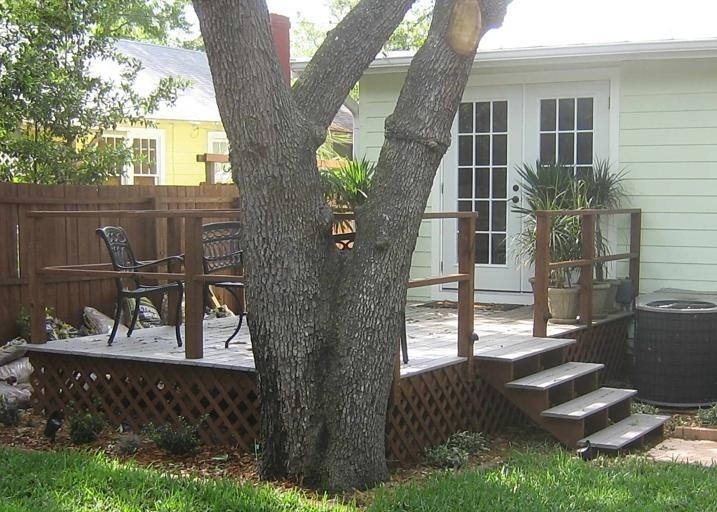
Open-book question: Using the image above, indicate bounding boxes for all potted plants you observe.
[502,158,628,323]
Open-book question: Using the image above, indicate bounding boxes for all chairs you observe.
[95,224,185,347]
[201,221,248,348]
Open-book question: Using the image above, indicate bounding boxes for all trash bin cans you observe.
[632,295,717,408]
